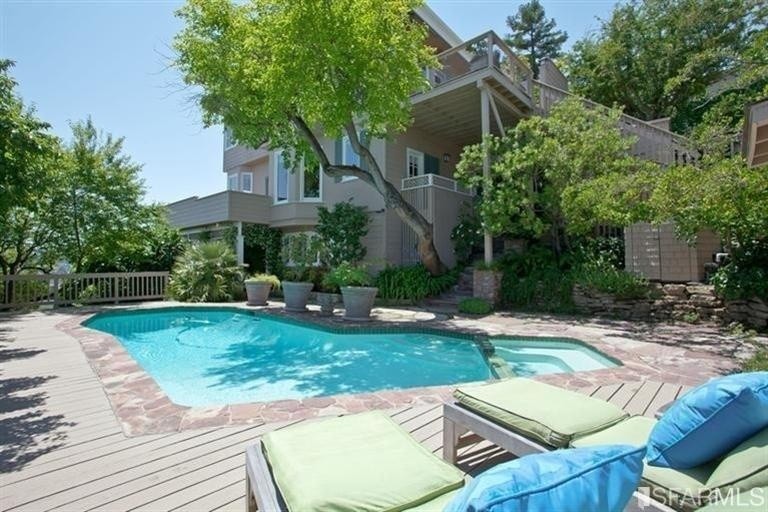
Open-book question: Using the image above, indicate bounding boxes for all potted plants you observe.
[317,280,338,315]
[244,274,280,306]
[334,265,379,322]
[282,268,314,312]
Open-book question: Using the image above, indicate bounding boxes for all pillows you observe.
[443,445,647,512]
[646,372,768,470]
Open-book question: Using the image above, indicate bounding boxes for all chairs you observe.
[246,408,469,512]
[442,376,768,512]
